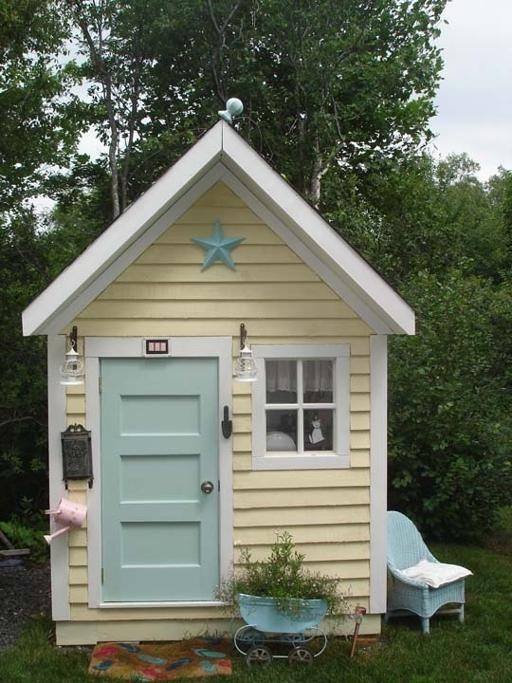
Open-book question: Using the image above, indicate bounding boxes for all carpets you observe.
[86,641,234,680]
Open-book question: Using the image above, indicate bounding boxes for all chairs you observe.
[384,510,474,636]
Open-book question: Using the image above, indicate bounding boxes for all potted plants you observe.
[212,529,359,634]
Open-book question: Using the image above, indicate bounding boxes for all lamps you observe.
[59,325,86,385]
[231,321,258,382]
